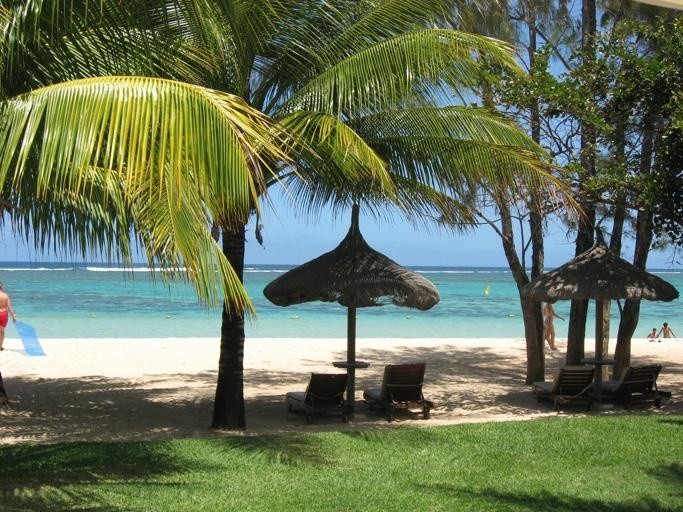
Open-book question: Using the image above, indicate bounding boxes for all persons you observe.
[543,320,551,347]
[0,281,16,351]
[647,328,660,337]
[543,299,565,350]
[657,322,675,338]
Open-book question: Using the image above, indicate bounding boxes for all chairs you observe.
[530,363,671,412]
[286,372,354,424]
[363,362,435,424]
